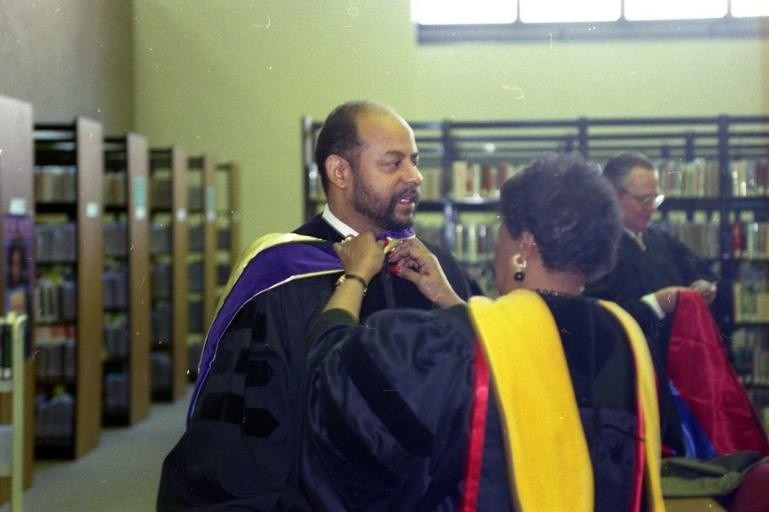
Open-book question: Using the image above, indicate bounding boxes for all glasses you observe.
[627,192,665,208]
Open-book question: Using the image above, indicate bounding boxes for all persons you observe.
[302,151,668,511]
[156,102,485,511]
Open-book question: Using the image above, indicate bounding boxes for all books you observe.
[101,144,128,409]
[309,151,443,247]
[729,157,769,414]
[452,159,527,301]
[592,158,721,278]
[149,151,233,390]
[27,125,77,438]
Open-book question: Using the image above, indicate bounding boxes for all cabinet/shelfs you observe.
[300,114,769,410]
